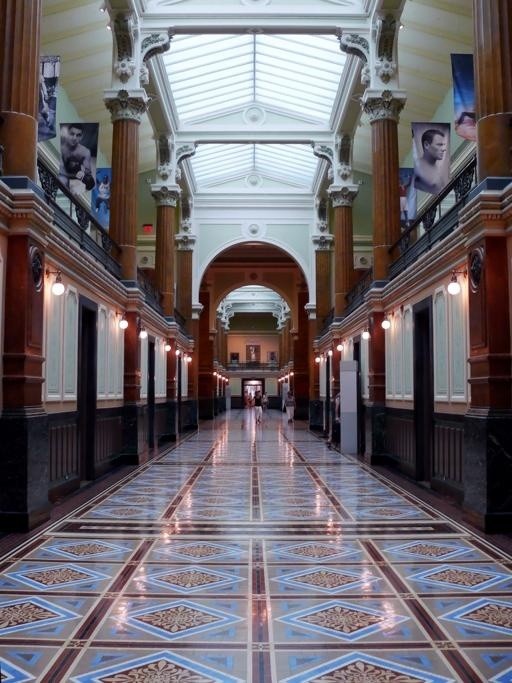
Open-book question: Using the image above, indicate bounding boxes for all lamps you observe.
[279,269,468,385]
[45,270,230,383]
[246,143,263,190]
[243,32,265,128]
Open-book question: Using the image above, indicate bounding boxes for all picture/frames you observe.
[245,345,260,360]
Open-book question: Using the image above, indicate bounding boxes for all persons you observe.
[61,124,95,195]
[325,390,341,451]
[399,175,412,228]
[285,391,296,423]
[413,129,449,196]
[95,175,111,214]
[246,389,269,424]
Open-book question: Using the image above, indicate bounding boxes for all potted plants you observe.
[231,355,237,364]
